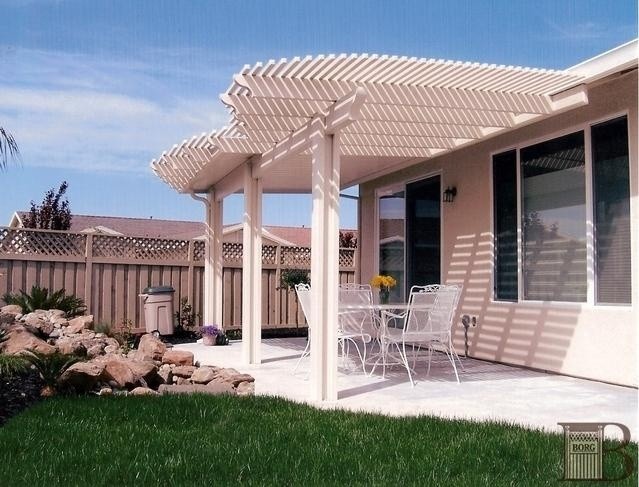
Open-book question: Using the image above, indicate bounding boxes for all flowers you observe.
[194,325,219,337]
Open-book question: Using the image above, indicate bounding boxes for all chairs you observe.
[295,284,465,386]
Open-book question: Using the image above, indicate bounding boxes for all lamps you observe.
[443,186,456,203]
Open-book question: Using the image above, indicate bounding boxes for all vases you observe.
[202,336,217,346]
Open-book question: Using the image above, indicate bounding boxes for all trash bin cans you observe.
[138,286,176,338]
